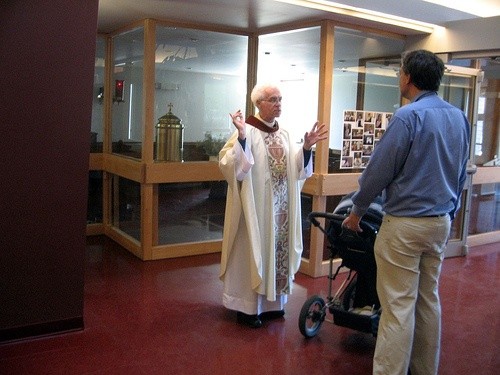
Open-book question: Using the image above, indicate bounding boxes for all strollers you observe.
[297,189,387,339]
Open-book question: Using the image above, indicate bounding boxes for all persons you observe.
[218,83,328,327]
[343,50,472,375]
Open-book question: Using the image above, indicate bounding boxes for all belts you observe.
[423,214,445,218]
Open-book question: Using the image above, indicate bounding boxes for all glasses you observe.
[261,98,282,103]
[396,71,405,77]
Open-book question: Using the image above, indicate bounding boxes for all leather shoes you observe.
[239,313,262,326]
[263,311,285,319]
